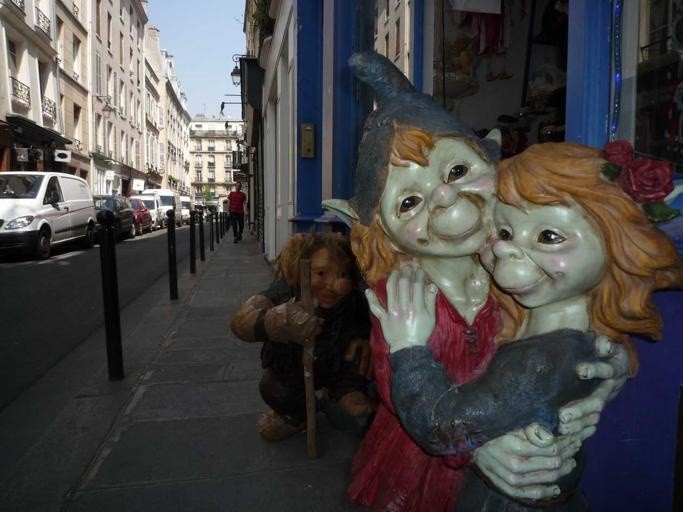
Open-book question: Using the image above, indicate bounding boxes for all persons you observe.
[319,46,638,511]
[100,189,123,243]
[228,223,383,447]
[224,181,249,244]
[363,135,682,511]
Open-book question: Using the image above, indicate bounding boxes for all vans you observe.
[142,188,183,228]
[0,170,99,259]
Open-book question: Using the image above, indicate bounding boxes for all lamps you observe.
[92,95,113,118]
[231,54,255,87]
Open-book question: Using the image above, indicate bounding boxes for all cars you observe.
[180,196,208,226]
[129,199,153,235]
[93,195,137,245]
[131,195,165,231]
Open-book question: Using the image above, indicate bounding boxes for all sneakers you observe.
[238,232,242,240]
[234,240,238,243]
